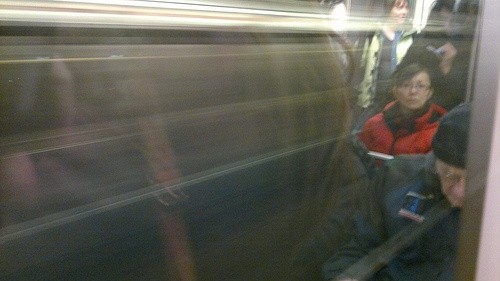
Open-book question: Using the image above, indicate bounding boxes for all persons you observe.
[232,0,483,280]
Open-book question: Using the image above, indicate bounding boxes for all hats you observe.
[429,123,468,167]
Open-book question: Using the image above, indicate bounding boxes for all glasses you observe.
[433,159,466,183]
